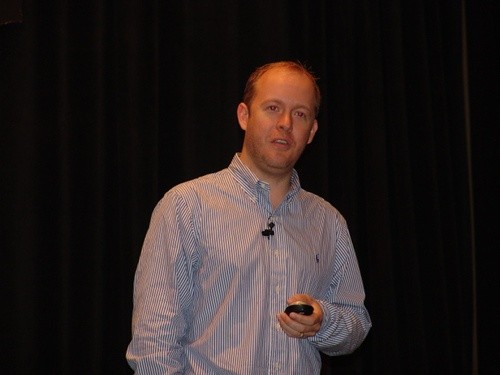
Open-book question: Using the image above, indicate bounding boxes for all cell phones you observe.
[284,304,314,316]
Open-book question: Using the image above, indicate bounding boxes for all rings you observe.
[299,331,304,339]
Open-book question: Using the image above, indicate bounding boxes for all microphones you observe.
[261,221,276,240]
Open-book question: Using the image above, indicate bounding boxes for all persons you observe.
[124,58,372,375]
